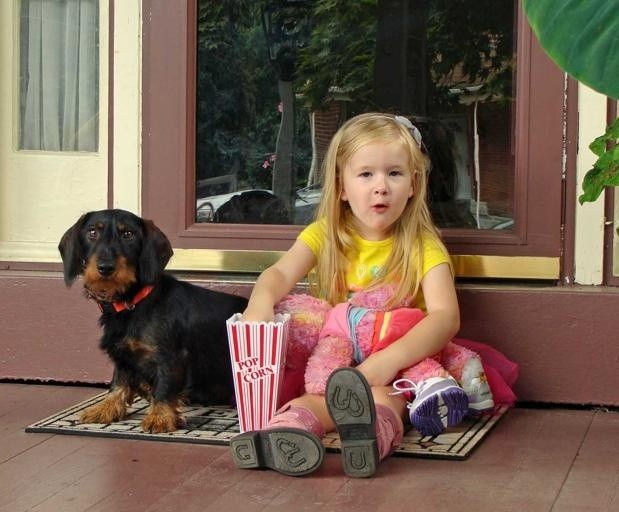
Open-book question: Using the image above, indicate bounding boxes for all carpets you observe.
[24,386,511,462]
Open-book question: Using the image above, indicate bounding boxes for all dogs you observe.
[57,208,250,435]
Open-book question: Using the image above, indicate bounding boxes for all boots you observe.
[326,368,401,479]
[230,405,325,476]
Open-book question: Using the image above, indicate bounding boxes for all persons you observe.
[228,111,463,479]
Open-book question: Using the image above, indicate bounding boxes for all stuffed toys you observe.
[275,285,519,435]
